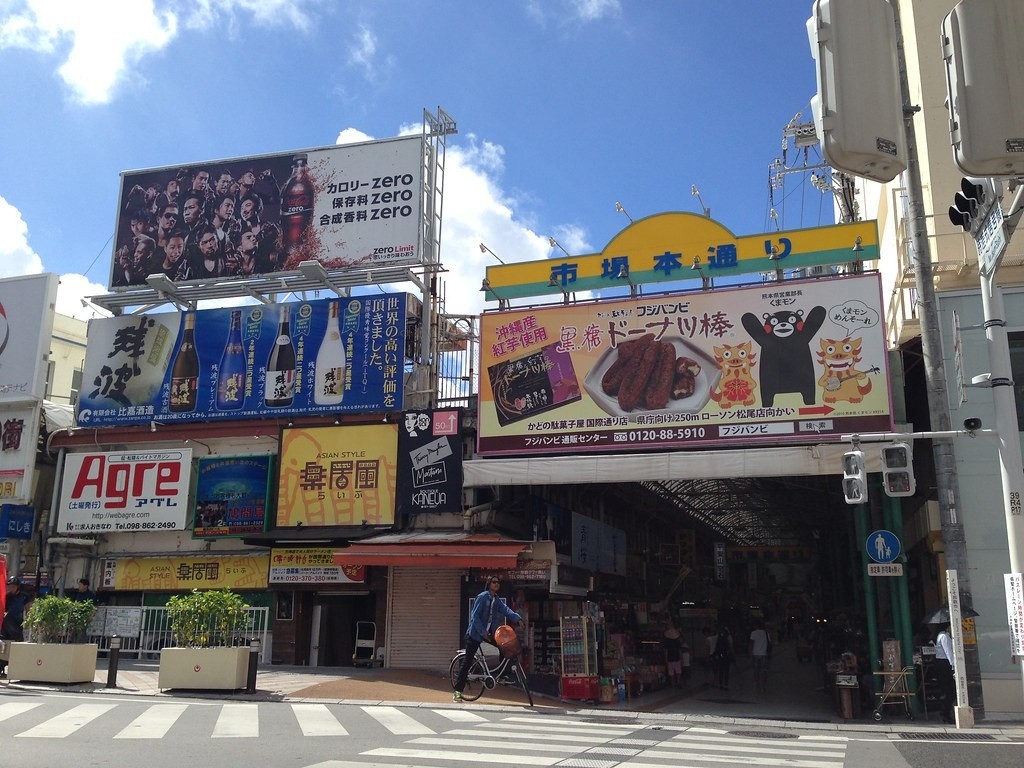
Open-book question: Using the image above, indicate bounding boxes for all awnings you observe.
[241,528,378,548]
[335,543,534,567]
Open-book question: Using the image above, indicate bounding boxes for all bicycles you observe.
[449,624,535,705]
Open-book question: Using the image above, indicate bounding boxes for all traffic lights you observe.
[881,445,917,497]
[843,450,870,504]
[947,174,1003,236]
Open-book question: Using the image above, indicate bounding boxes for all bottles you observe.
[278,154,314,261]
[314,302,346,406]
[217,310,247,410]
[169,313,200,413]
[534,619,595,675]
[264,305,295,407]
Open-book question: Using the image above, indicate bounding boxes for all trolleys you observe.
[353,619,387,669]
[871,664,922,722]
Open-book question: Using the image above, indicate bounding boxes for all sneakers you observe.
[452,691,463,703]
[497,676,517,685]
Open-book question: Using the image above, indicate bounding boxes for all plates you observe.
[582,335,723,423]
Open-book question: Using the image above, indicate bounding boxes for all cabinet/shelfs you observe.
[534,619,561,676]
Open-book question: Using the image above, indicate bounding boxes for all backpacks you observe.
[495,624,519,656]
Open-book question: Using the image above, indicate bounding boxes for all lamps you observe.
[146,273,191,309]
[280,279,303,301]
[361,520,367,529]
[81,299,108,318]
[296,519,302,528]
[366,270,387,294]
[477,184,863,310]
[66,411,391,447]
[297,259,347,298]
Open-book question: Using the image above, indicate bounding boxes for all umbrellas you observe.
[921,604,980,624]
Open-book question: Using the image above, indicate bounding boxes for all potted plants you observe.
[6,596,99,680]
[159,586,250,691]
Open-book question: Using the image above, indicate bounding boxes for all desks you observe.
[624,672,633,703]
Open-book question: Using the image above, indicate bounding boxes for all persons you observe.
[71,578,97,643]
[452,576,523,703]
[748,620,771,694]
[663,628,683,688]
[934,622,955,724]
[2,576,30,641]
[114,168,282,286]
[701,625,733,688]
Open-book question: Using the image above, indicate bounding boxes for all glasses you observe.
[491,580,501,584]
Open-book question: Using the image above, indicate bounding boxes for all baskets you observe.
[499,635,524,659]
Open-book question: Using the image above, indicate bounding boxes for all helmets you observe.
[7,576,21,586]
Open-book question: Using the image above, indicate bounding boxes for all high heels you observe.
[942,716,956,724]
